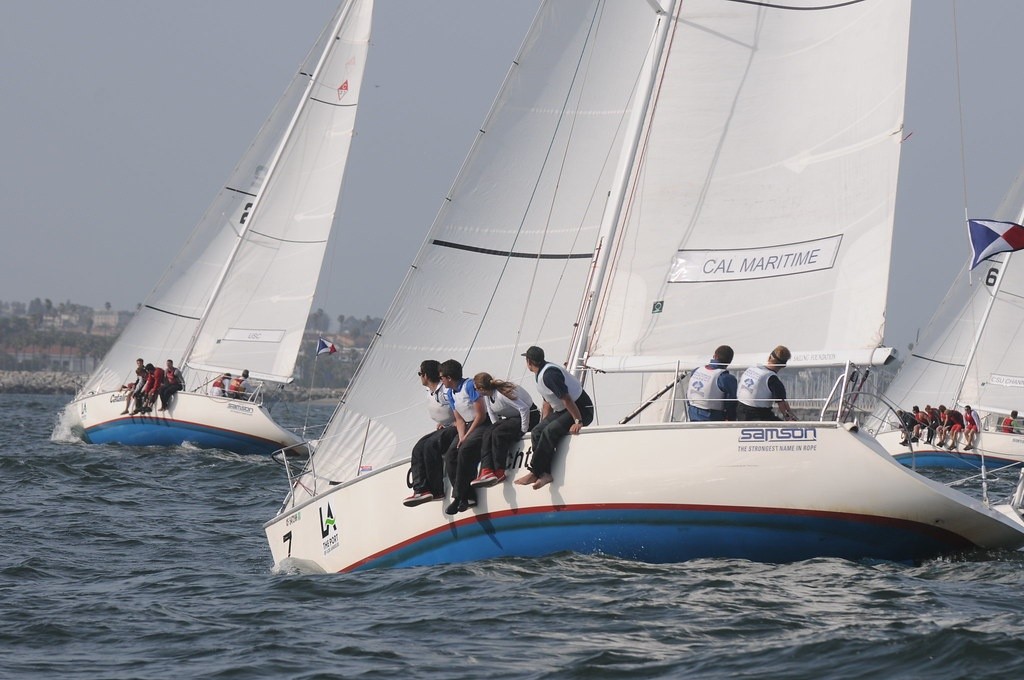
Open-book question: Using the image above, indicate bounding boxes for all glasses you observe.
[438,376,444,380]
[474,388,478,391]
[418,372,423,376]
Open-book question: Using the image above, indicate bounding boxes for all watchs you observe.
[575,419,582,424]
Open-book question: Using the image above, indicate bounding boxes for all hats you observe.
[521,346,544,361]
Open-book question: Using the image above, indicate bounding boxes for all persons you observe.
[121,358,185,415]
[213,373,232,397]
[403,359,457,507]
[963,405,980,451]
[736,346,793,421]
[228,370,256,401]
[514,346,594,490]
[438,359,492,515]
[686,344,738,422]
[897,405,965,450]
[1001,410,1021,434]
[470,372,541,488]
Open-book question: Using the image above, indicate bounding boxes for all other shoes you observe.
[121,407,167,416]
[899,436,972,451]
[445,499,468,514]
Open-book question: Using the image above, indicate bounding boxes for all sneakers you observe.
[470,469,506,488]
[402,491,445,507]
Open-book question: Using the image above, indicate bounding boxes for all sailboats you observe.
[51,0,373,466]
[854,212,1024,476]
[256,0,1024,583]
